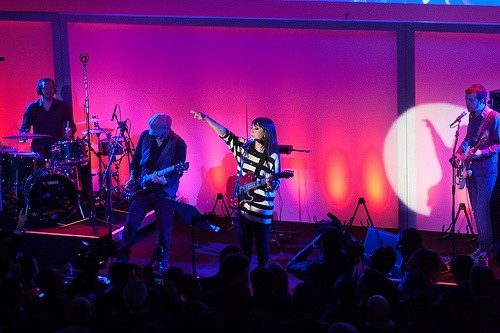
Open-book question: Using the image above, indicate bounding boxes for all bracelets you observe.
[312,242,319,249]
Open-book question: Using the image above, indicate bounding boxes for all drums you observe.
[52,139,88,165]
[24,167,78,223]
[0,145,18,187]
[55,165,82,189]
[99,139,124,156]
[4,152,35,190]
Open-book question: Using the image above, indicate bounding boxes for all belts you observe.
[471,156,493,162]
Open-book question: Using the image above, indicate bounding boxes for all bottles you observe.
[480,256,488,267]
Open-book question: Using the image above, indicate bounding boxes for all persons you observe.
[190,109,281,267]
[449,83,500,260]
[115,113,186,277]
[0,232,500,333]
[19,78,76,169]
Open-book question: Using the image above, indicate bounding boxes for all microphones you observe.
[242,138,254,147]
[111,107,117,121]
[450,112,466,127]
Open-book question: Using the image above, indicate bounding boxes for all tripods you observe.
[72,64,130,221]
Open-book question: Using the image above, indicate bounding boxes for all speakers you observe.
[363,227,400,261]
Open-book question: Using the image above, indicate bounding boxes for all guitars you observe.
[456,131,488,191]
[125,161,190,200]
[226,169,294,208]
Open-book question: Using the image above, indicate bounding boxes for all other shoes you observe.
[478,252,488,258]
[153,267,169,276]
[472,249,482,256]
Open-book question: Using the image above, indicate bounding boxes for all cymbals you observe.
[82,128,115,134]
[4,134,53,140]
[76,119,111,124]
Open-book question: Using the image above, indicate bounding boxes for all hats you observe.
[149,114,171,135]
